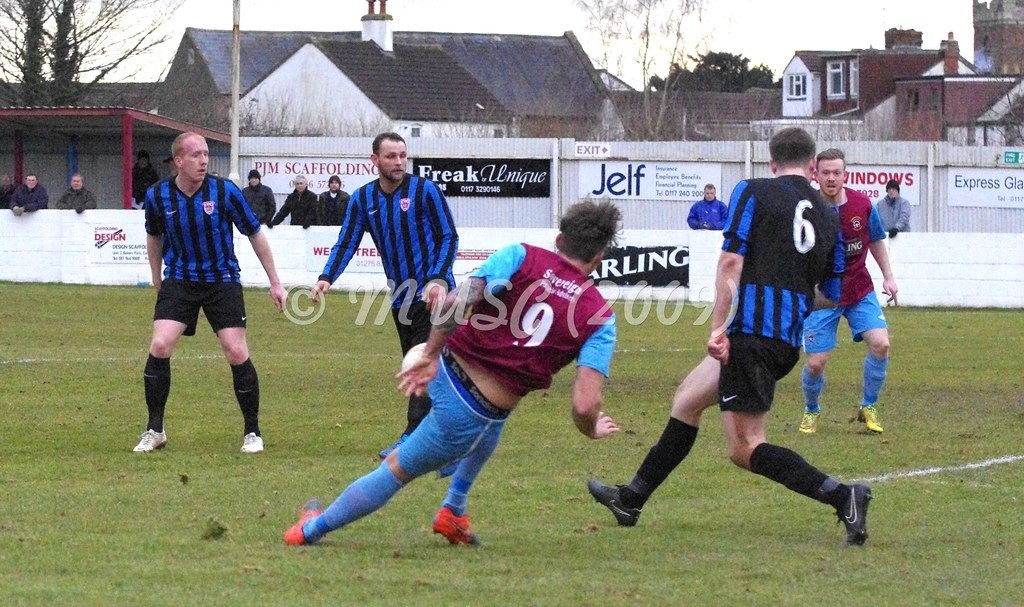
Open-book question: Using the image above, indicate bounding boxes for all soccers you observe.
[401,342,429,397]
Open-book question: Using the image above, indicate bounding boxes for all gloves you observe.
[13,205,25,216]
[76,207,82,213]
[698,221,712,230]
[888,228,898,238]
[267,221,273,228]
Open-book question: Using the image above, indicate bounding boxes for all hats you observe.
[248,169,261,180]
[886,179,900,193]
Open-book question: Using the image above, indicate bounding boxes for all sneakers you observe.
[798,411,821,433]
[133,429,168,453]
[284,499,325,546]
[857,405,883,435]
[240,432,264,454]
[587,480,643,527]
[432,507,481,546]
[833,483,873,546]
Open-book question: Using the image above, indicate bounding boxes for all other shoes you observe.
[378,433,409,458]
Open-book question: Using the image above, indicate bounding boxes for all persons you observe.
[0,171,21,209]
[318,176,350,227]
[267,175,317,229]
[286,197,622,547]
[798,148,897,435]
[586,126,874,548]
[132,132,288,454]
[310,132,459,460]
[163,157,178,176]
[132,149,159,210]
[687,184,729,230]
[874,180,911,238]
[9,174,48,216]
[241,170,276,224]
[55,174,97,213]
